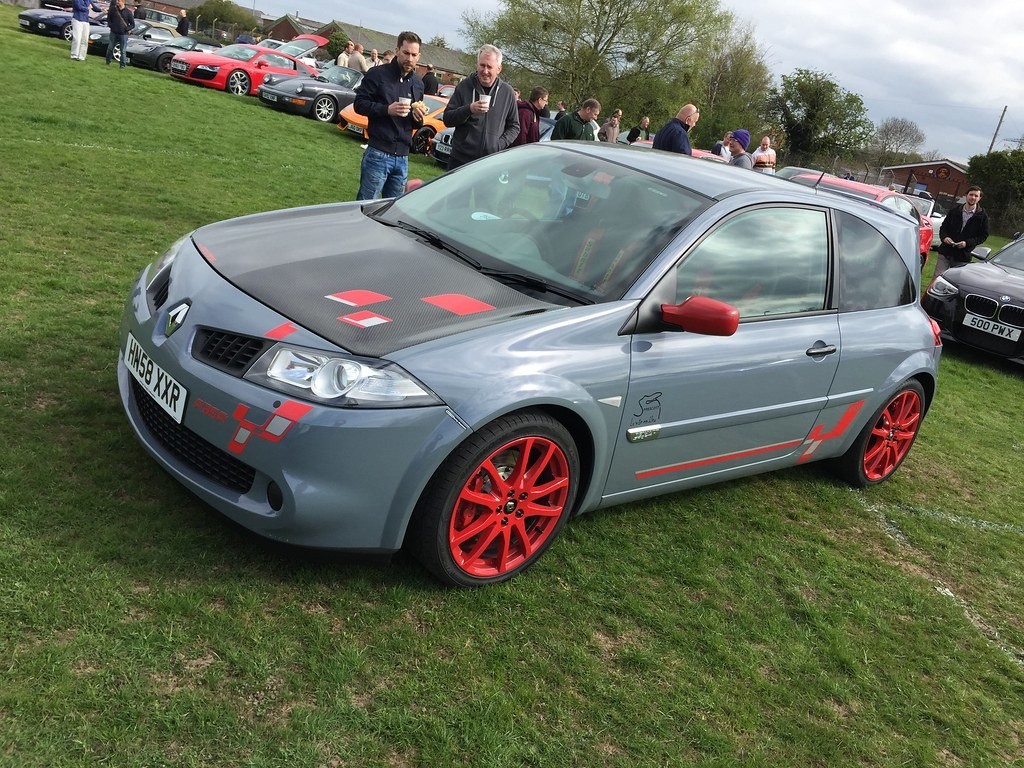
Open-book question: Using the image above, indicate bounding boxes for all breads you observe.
[411,100,425,109]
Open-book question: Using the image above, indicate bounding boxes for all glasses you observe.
[537,96,548,105]
[728,137,739,142]
[348,43,354,47]
[689,107,699,117]
[618,114,622,116]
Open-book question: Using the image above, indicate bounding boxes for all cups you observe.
[398,97,411,117]
[480,94,491,112]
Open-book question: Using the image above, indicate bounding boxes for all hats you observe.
[731,129,751,151]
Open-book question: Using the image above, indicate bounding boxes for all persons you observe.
[133,0,146,19]
[70,0,106,61]
[727,129,753,169]
[105,0,135,68]
[712,130,733,161]
[752,135,776,175]
[627,116,650,144]
[176,10,189,36]
[509,86,550,147]
[554,100,566,120]
[844,172,854,180]
[933,186,989,279]
[551,99,601,141]
[422,64,438,95]
[334,41,395,73]
[597,109,622,143]
[889,185,897,192]
[442,44,520,209]
[652,103,700,155]
[353,31,428,200]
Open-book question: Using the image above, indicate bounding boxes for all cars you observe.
[548,111,603,142]
[788,174,934,274]
[429,115,556,168]
[337,91,452,156]
[15,6,336,74]
[437,83,457,98]
[169,35,329,96]
[774,166,837,180]
[902,194,948,247]
[921,231,1024,363]
[117,140,945,589]
[629,140,731,164]
[257,66,367,124]
[615,132,656,146]
